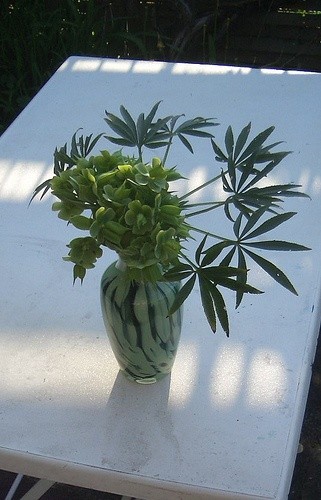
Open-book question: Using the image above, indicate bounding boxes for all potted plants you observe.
[27,99,311,384]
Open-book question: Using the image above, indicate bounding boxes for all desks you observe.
[0,55,321,499]
